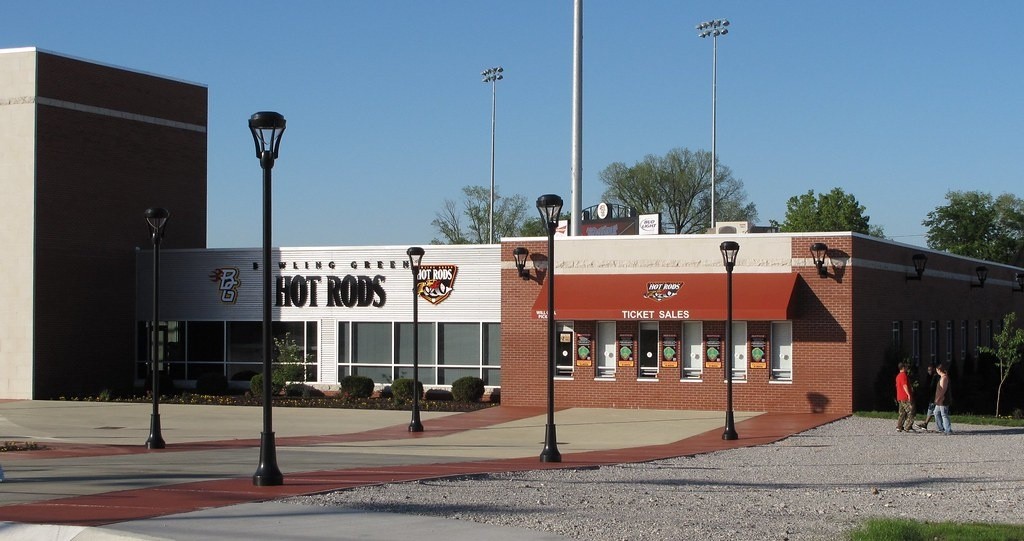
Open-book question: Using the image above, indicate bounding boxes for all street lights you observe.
[535,194,562,464]
[145,204,170,450]
[695,15,731,228]
[719,240,740,440]
[481,65,503,245]
[406,246,426,432]
[248,110,288,486]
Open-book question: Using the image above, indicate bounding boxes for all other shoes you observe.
[935,429,944,434]
[914,424,927,431]
[896,427,904,432]
[904,427,916,433]
[944,431,954,435]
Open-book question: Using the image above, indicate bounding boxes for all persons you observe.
[934,364,953,434]
[916,365,940,430]
[895,362,914,432]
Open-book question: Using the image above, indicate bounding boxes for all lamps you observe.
[810,244,839,277]
[1011,275,1024,292]
[907,254,927,281]
[513,247,530,277]
[971,266,989,288]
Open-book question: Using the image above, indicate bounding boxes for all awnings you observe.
[532,272,801,320]
